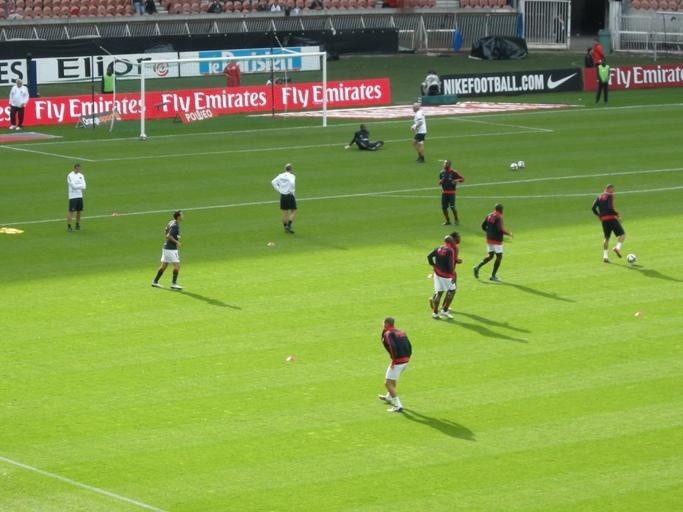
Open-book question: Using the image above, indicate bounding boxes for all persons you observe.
[376,314,413,412]
[310,0,324,10]
[221,58,242,87]
[101,66,117,94]
[585,48,594,68]
[269,162,299,234]
[592,38,604,64]
[595,58,610,103]
[436,157,464,226]
[148,208,186,290]
[66,164,87,232]
[343,122,385,151]
[145,0,157,15]
[208,0,222,13]
[589,183,626,264]
[425,230,463,320]
[409,100,429,163]
[472,202,515,283]
[264,1,271,11]
[7,77,29,131]
[256,1,264,10]
[133,0,145,15]
[271,1,280,12]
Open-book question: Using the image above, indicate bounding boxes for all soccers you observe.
[510,163,518,171]
[139,134,147,141]
[626,254,636,263]
[518,161,525,168]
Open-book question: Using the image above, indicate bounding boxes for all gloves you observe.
[345,146,350,149]
[412,125,416,131]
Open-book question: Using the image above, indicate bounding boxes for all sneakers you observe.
[454,217,460,225]
[387,406,403,412]
[613,248,621,258]
[171,284,182,289]
[152,282,163,287]
[429,266,501,319]
[417,158,424,163]
[76,222,80,229]
[442,221,451,225]
[285,227,289,232]
[604,257,608,262]
[68,224,72,231]
[288,227,295,233]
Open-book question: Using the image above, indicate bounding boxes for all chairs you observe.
[460,0,507,9]
[0,0,134,20]
[405,0,436,9]
[161,0,316,15]
[633,0,683,12]
[322,0,375,9]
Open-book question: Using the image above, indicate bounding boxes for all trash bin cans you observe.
[597,29,612,56]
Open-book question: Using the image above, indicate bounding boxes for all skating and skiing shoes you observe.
[379,395,391,403]
